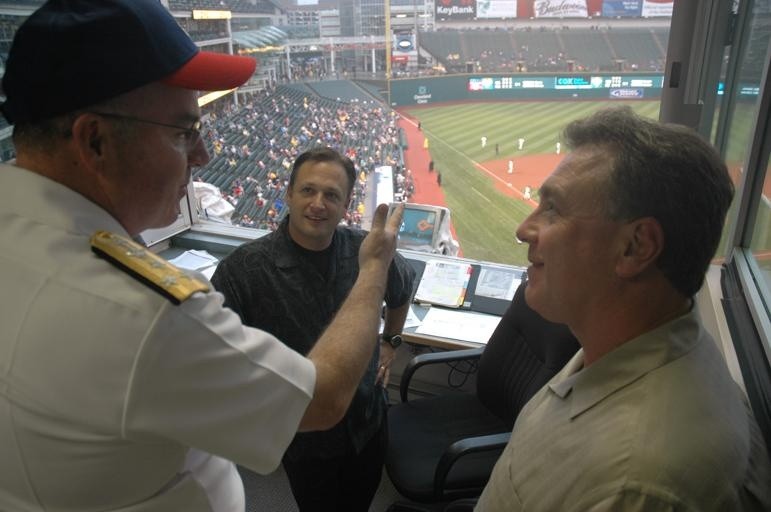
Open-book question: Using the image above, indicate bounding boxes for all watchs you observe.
[381,333,403,348]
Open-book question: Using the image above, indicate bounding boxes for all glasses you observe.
[63,112,204,146]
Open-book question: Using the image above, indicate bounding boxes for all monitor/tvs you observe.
[386,204,441,251]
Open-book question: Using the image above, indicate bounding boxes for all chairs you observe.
[384,281,582,512]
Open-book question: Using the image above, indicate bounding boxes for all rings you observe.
[380,367,385,370]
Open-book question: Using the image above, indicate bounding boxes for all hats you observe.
[1,0,258,124]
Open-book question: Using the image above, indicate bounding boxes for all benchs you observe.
[190,85,379,233]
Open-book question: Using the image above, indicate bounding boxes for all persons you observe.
[182,24,666,231]
[206,148,415,512]
[469,106,771,512]
[0,0,406,512]
